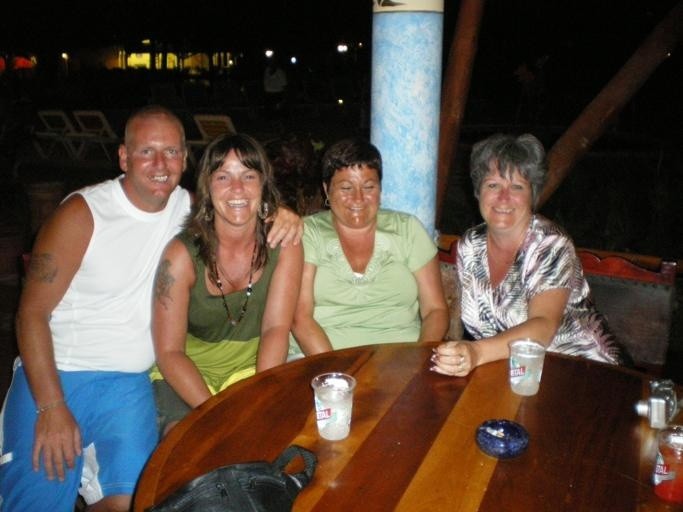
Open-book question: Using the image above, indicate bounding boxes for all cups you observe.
[311,372,356,440]
[508,338,544,396]
[650,379,678,429]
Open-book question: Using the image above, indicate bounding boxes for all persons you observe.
[288,138,450,361]
[429,134,640,377]
[147,133,305,439]
[0,108,305,512]
[262,133,324,220]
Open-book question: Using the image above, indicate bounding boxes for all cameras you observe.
[635,379,677,429]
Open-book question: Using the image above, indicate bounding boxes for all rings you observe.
[459,353,465,365]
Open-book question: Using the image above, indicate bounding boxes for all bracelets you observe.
[36,402,65,414]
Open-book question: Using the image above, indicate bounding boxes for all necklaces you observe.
[214,237,257,326]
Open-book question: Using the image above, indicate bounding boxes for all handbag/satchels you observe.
[143,444,318,512]
